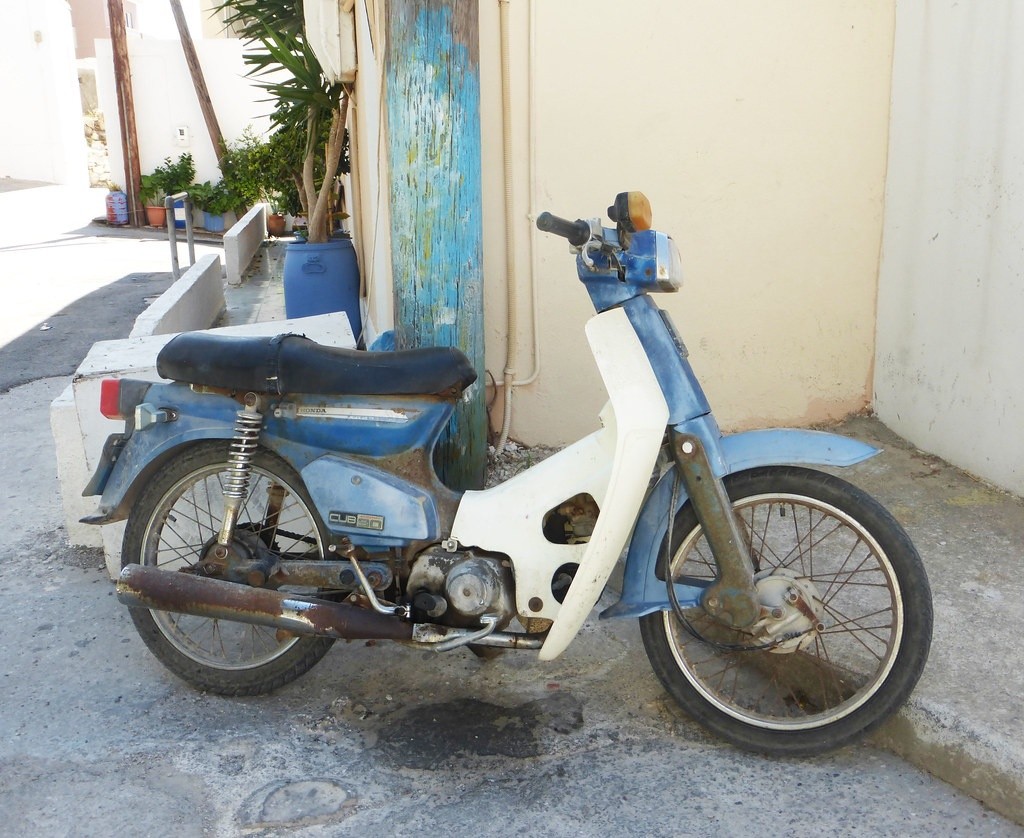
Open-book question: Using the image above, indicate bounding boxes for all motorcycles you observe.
[75,189,934,760]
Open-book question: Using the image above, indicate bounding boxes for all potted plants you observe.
[106,0,364,345]
